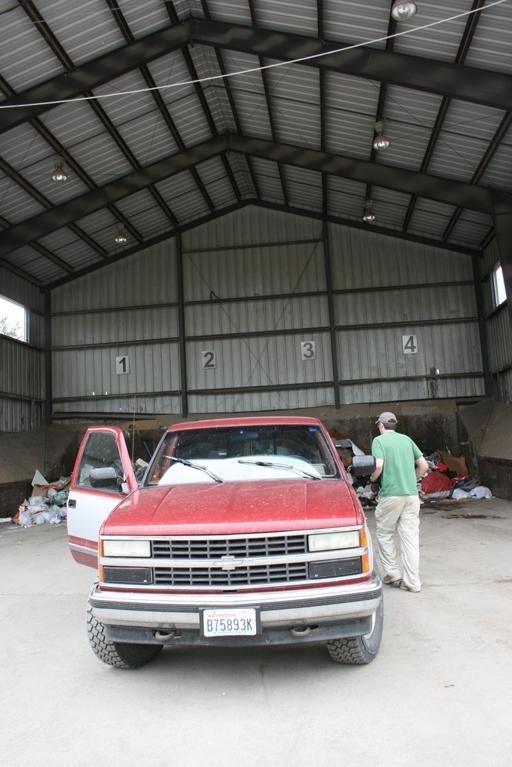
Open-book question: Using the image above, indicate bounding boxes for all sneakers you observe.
[398,580,420,592]
[380,569,402,584]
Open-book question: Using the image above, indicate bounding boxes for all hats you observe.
[374,410,400,425]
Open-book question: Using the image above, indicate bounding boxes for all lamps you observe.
[363,199,376,221]
[373,120,390,151]
[391,0,417,21]
[52,156,67,181]
[115,223,127,243]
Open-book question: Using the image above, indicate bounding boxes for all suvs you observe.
[60,411,386,670]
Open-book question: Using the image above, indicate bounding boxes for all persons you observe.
[370,411,428,593]
